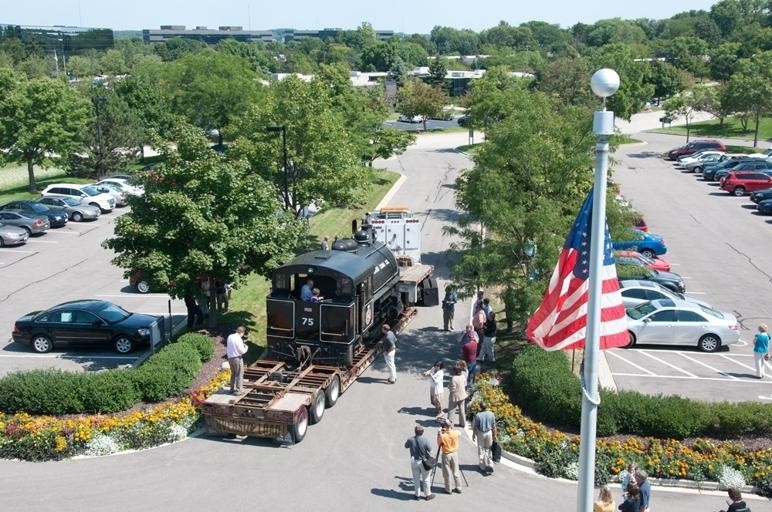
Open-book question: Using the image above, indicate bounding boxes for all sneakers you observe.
[415,496,420,501]
[426,494,435,500]
[447,488,461,494]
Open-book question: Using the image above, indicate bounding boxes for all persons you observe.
[226,325,248,396]
[520,235,537,279]
[184,275,236,328]
[321,234,340,253]
[381,323,399,384]
[405,425,436,501]
[503,284,514,335]
[422,284,498,428]
[472,400,498,476]
[437,418,462,495]
[752,324,772,378]
[594,485,615,512]
[618,463,651,511]
[301,279,324,304]
[720,488,746,512]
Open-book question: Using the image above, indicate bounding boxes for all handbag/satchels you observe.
[422,456,437,470]
[491,441,501,462]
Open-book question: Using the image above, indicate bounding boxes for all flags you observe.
[524,189,632,353]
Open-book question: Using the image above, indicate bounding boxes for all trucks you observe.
[199,204,438,442]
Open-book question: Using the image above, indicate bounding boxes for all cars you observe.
[677,148,772,216]
[0,175,145,247]
[399,113,425,123]
[130,265,233,301]
[11,299,162,355]
[668,140,726,161]
[619,298,741,352]
[612,193,686,310]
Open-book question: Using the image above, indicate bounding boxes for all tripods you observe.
[431,429,468,490]
